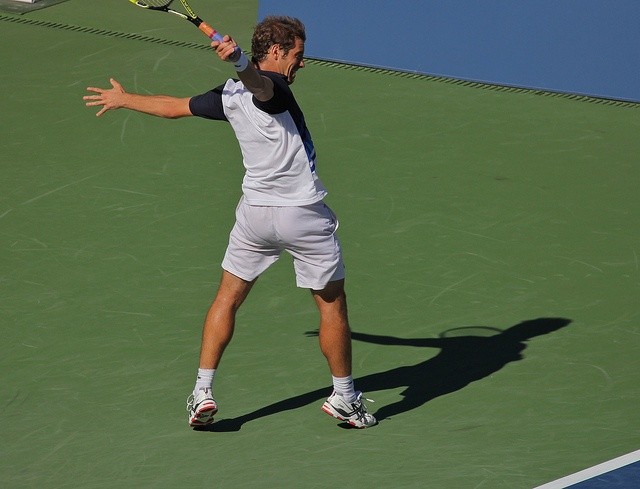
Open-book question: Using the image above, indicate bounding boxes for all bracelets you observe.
[231,51,249,73]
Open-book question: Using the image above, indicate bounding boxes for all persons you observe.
[82,14,379,430]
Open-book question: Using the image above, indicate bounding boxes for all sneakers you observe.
[186,386,218,427]
[321,390,377,428]
